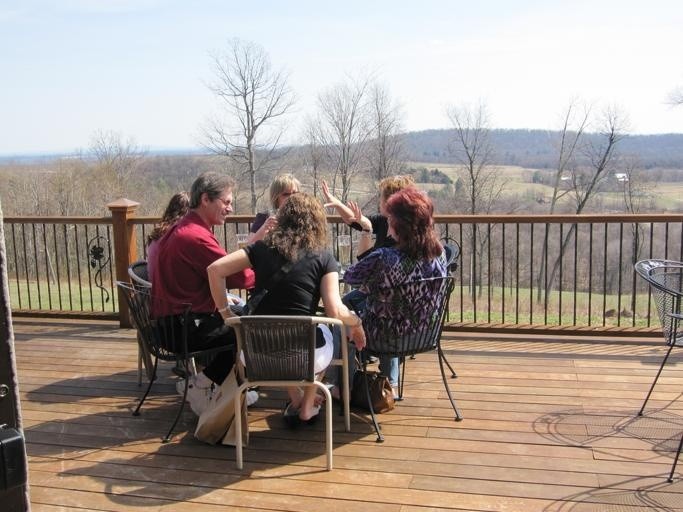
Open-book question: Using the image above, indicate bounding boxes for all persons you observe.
[144,173,449,429]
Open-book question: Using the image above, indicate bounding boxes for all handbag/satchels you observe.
[351,370,396,414]
[194,363,249,448]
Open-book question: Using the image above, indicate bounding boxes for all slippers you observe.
[284,398,322,426]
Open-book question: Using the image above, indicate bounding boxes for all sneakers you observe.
[331,386,341,401]
[173,359,259,417]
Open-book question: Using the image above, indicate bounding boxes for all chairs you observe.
[128,260,243,388]
[118,281,234,443]
[224,314,352,471]
[438,236,463,379]
[348,275,463,443]
[632,257,683,483]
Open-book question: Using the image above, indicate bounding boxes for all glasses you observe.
[214,194,233,208]
[281,191,299,197]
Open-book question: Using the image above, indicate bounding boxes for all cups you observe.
[235,233,249,248]
[336,235,353,276]
